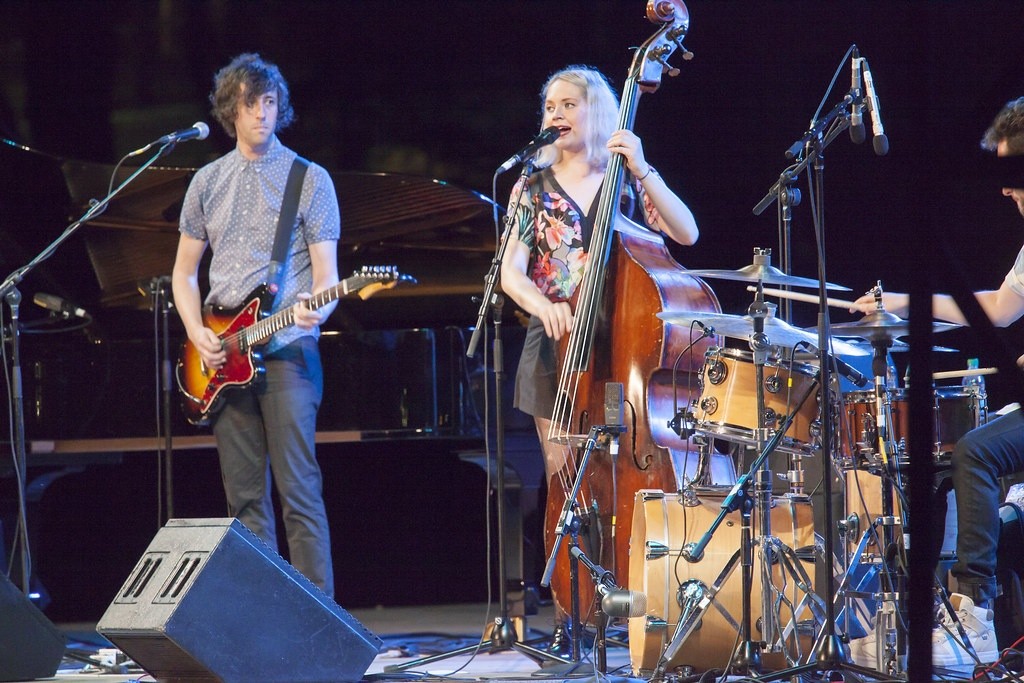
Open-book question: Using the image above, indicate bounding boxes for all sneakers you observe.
[930,593,998,665]
[849,602,896,672]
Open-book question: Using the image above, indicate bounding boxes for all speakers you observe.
[95,518,386,683]
[0,573,66,683]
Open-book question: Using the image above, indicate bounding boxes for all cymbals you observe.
[796,311,966,354]
[680,262,856,293]
[653,309,871,359]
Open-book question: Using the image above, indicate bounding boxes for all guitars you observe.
[177,262,419,427]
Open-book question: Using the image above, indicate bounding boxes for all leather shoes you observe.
[547,625,572,660]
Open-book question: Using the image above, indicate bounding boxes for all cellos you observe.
[538,0,726,673]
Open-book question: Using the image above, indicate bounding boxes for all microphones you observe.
[862,59,888,155]
[850,48,866,144]
[872,356,887,441]
[800,340,868,387]
[496,126,560,175]
[149,122,209,147]
[33,292,92,319]
[603,382,622,463]
[602,591,647,617]
[698,320,714,338]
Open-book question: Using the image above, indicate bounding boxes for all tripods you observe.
[384,90,993,683]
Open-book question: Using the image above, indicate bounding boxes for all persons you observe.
[500,64,700,654]
[171,53,339,600]
[848,97,1024,666]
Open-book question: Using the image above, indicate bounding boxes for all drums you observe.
[839,384,986,469]
[625,488,816,677]
[841,472,983,564]
[693,342,831,459]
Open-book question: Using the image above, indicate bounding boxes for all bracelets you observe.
[637,169,651,181]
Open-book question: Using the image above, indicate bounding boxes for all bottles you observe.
[962,358,988,417]
